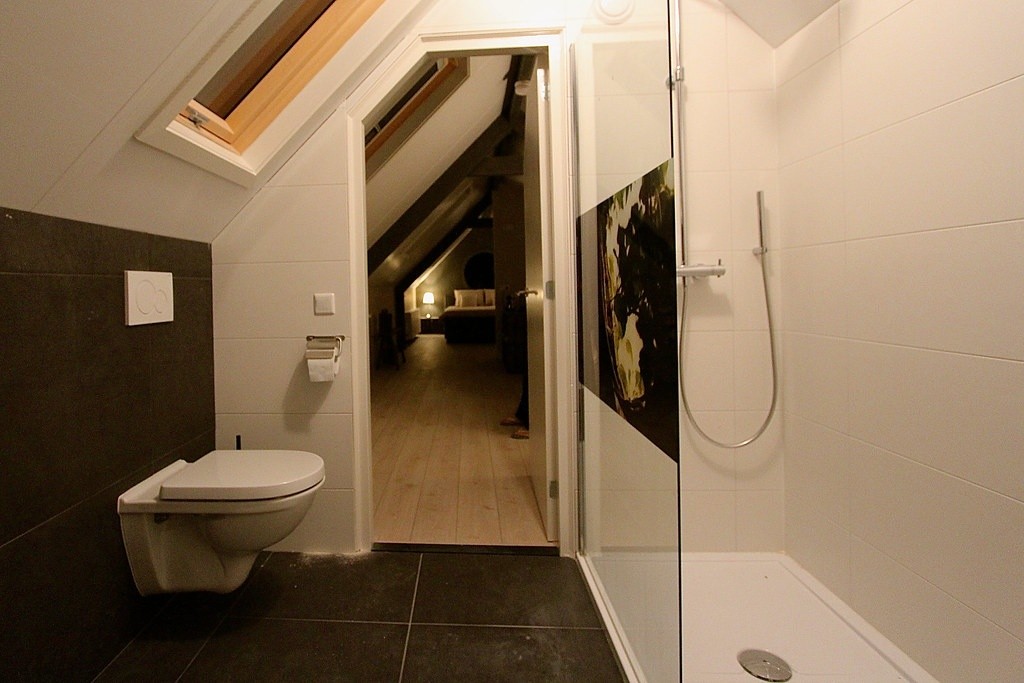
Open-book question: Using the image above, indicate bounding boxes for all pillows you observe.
[454,290,483,306]
[460,293,477,307]
[484,289,495,306]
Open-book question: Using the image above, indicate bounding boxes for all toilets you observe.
[116,449,326,598]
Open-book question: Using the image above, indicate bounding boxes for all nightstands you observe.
[420,315,440,333]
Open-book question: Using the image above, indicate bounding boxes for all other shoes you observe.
[511,427,529,439]
[500,415,520,425]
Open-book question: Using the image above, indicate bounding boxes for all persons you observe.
[500,372,530,439]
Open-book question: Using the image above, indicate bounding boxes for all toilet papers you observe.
[306,347,340,382]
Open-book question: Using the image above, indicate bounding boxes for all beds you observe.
[439,291,496,345]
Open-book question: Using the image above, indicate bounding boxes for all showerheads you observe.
[757,190,765,243]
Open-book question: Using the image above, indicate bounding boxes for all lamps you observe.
[422,292,434,318]
